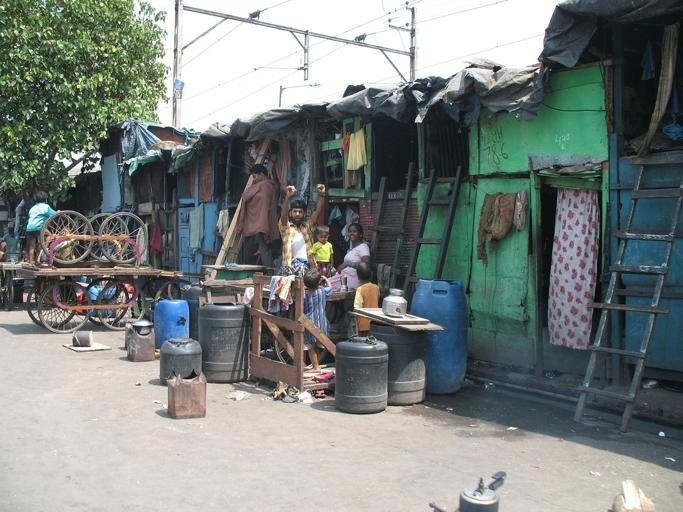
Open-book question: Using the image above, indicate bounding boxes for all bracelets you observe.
[318,194,325,197]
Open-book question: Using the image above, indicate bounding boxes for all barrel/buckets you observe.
[197,304,252,382]
[409,278,470,395]
[73,331,93,347]
[153,296,190,349]
[13,277,25,304]
[370,320,427,406]
[336,335,389,414]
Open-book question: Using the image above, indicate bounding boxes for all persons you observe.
[297,268,334,372]
[237,164,280,272]
[25,191,61,265]
[353,262,380,336]
[321,222,371,294]
[310,222,334,272]
[276,181,327,276]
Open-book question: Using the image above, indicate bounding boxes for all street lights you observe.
[278,83,321,108]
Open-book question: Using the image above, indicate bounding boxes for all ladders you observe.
[574,150,683,432]
[402,163,466,312]
[366,161,415,308]
